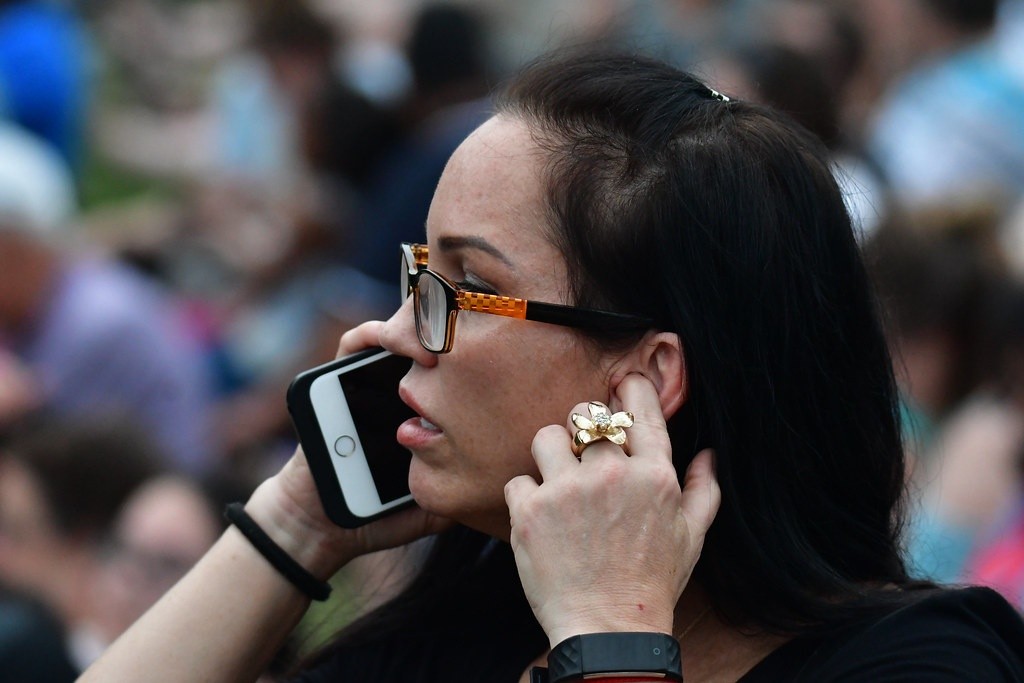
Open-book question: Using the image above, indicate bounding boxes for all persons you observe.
[0,1,1024,683]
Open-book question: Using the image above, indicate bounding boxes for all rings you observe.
[571,401,634,457]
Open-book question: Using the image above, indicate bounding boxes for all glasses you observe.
[398,243,668,354]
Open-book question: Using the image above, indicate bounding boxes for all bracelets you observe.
[227,503,333,604]
[530,631,684,683]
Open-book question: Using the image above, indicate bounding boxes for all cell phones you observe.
[285,345,414,528]
